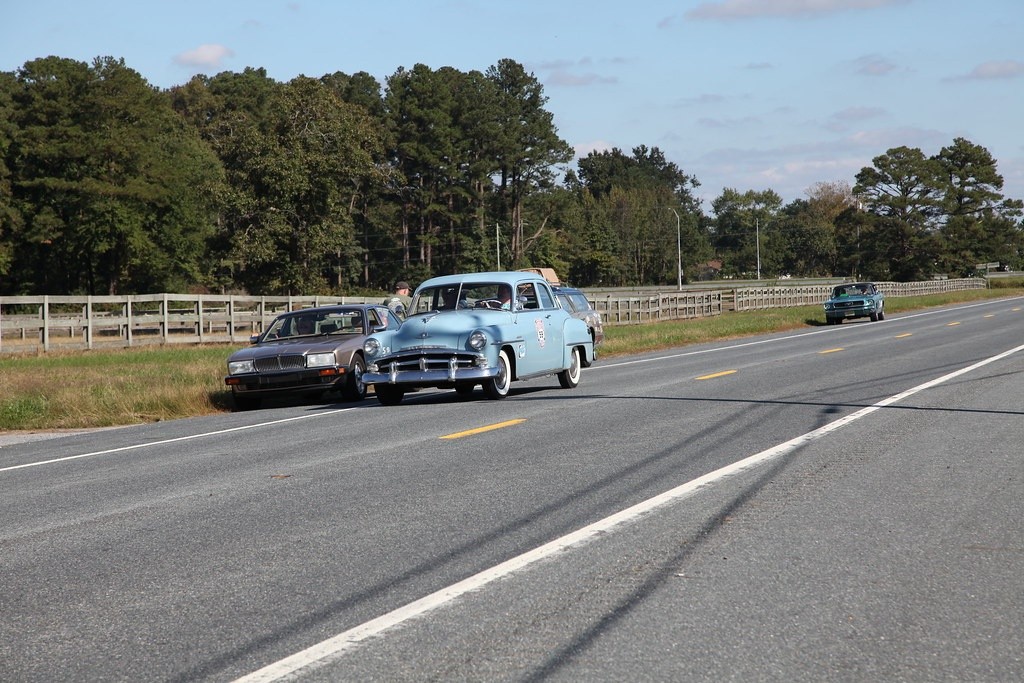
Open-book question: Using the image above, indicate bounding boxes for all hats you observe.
[396,282,413,292]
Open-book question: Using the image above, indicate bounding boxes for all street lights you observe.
[668,208,682,290]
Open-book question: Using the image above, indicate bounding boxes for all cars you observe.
[823,282,884,325]
[361,272,595,406]
[225,303,422,406]
[521,288,606,368]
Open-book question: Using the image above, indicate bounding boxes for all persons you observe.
[474,284,521,310]
[440,291,457,309]
[382,280,412,321]
[838,288,847,296]
[296,318,313,334]
[861,288,868,294]
